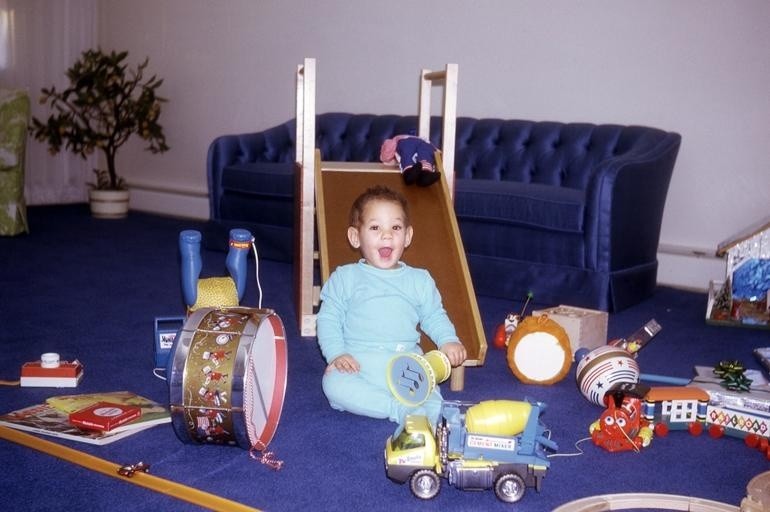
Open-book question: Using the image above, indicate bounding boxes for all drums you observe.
[170,307,289,451]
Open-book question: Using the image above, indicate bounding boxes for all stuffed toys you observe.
[377,133,442,187]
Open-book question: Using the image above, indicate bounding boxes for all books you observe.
[0,402,159,446]
[45,388,172,435]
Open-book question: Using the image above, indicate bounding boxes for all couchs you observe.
[206,111,684,316]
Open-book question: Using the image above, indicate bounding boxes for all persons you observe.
[313,187,468,426]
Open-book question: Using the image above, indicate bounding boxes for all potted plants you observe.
[28,45,173,220]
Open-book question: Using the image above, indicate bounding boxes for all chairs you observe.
[0,87,33,236]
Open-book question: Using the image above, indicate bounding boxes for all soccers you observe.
[576,345,641,408]
